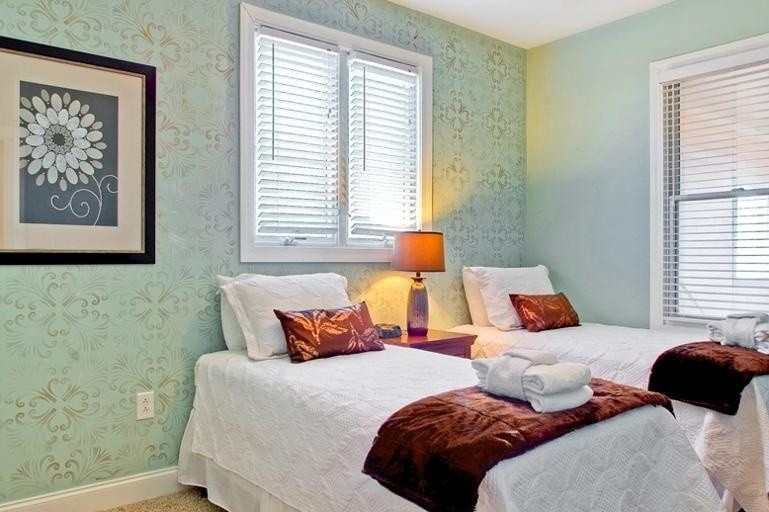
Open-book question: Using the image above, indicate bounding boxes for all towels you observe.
[707,310,768,354]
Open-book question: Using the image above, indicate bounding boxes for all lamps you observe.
[389,229,447,337]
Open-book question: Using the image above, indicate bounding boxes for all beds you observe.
[447,321,769,512]
[176,345,726,511]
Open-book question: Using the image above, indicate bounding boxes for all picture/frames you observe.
[0,35,158,265]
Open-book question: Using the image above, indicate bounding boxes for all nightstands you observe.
[384,326,478,359]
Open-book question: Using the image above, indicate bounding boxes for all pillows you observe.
[459,265,581,331]
[214,271,384,365]
